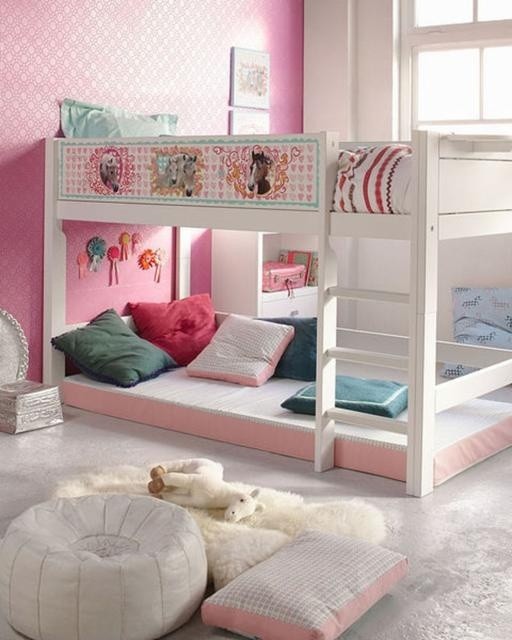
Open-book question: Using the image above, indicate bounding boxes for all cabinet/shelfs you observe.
[210,229,321,320]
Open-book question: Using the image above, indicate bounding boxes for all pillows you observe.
[202,532,409,640]
[281,374,408,418]
[259,318,317,382]
[61,98,179,137]
[51,306,177,388]
[185,312,295,387]
[440,283,511,381]
[126,293,216,367]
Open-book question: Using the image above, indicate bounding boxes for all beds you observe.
[36,127,511,498]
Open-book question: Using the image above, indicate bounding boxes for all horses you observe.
[158,154,197,197]
[100,153,119,192]
[247,150,275,194]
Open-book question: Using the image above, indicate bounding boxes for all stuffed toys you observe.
[149,456,266,522]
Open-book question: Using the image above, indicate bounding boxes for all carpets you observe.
[55,468,387,592]
